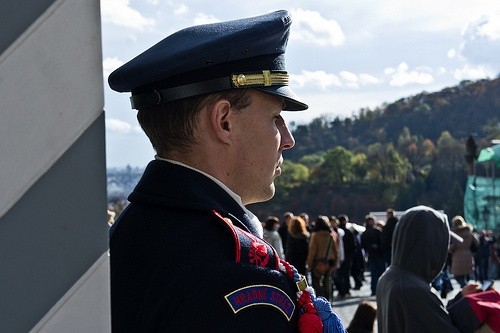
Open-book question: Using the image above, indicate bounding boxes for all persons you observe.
[108,10,348,333]
[263,208,500,306]
[107,203,124,227]
[377,205,500,333]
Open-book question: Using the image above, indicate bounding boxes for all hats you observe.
[108,10,309,111]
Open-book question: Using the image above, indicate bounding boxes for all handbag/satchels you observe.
[313,261,329,278]
[469,235,480,253]
